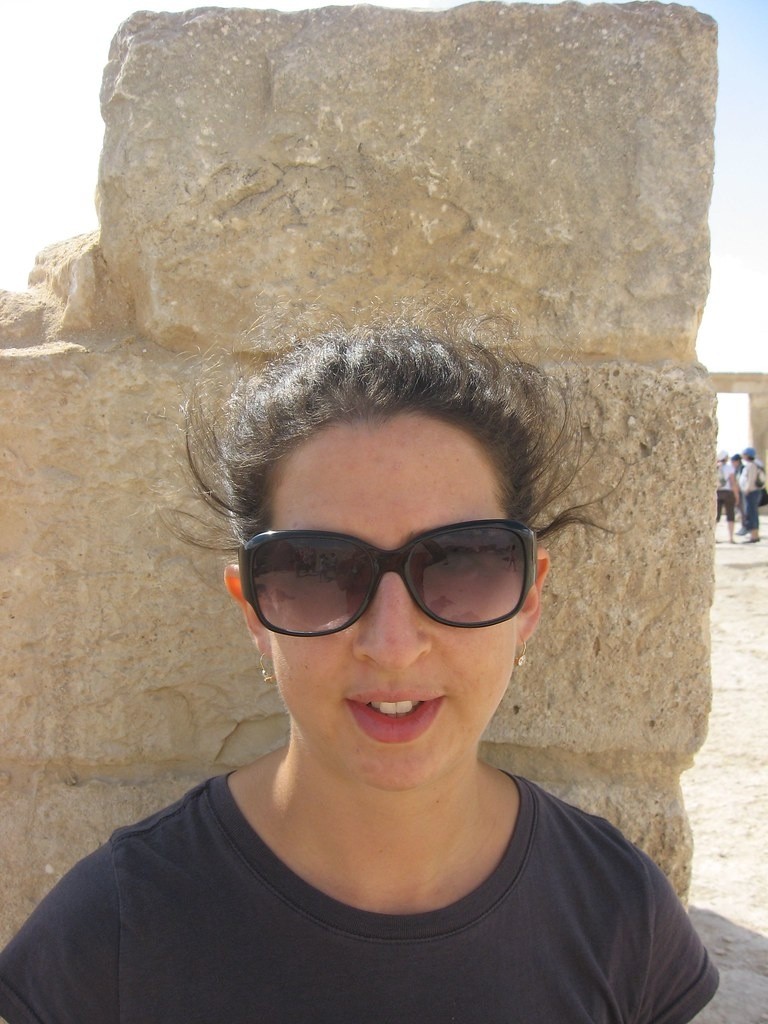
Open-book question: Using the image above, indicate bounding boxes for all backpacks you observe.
[716,464,728,486]
[755,464,766,488]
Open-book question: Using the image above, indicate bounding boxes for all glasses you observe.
[239,518,536,638]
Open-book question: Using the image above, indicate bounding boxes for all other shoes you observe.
[735,528,749,535]
[745,538,760,543]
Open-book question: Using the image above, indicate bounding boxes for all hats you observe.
[717,451,727,461]
[730,454,740,461]
[743,449,756,460]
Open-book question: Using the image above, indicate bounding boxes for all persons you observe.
[718,448,762,543]
[2,321,720,1024]
[507,545,517,572]
[293,548,338,584]
